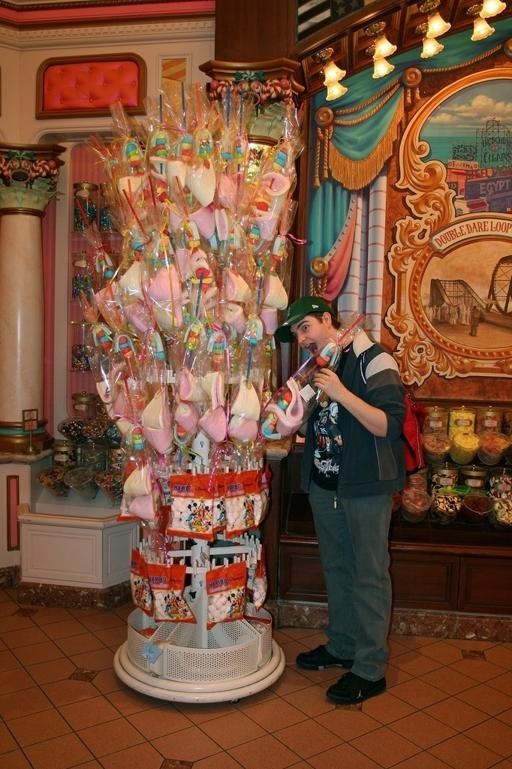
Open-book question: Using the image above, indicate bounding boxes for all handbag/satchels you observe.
[401,387,424,476]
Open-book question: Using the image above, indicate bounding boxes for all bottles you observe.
[36,388,124,506]
[74,179,122,301]
[389,463,511,530]
[419,406,511,467]
[69,319,91,373]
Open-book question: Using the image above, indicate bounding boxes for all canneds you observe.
[53,446,70,466]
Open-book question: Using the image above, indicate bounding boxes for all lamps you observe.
[304,0,510,102]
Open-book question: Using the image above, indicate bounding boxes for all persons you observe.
[274,296,407,704]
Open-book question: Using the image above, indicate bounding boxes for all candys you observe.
[77,85,305,541]
[205,562,247,630]
[147,564,197,623]
[252,543,268,612]
[130,548,153,617]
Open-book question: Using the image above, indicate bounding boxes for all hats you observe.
[275,296,336,343]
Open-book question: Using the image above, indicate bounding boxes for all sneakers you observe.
[296,645,354,670]
[326,671,386,705]
